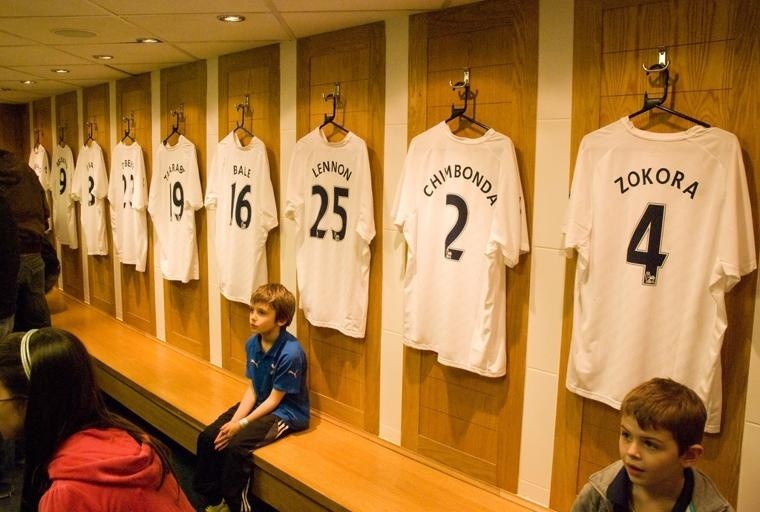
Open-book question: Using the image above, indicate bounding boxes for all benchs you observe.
[36,282,560,512]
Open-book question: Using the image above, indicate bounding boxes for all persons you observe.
[0,147,61,499]
[0,327,199,512]
[190,282,310,511]
[569,376,738,511]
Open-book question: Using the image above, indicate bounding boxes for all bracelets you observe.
[240,418,249,428]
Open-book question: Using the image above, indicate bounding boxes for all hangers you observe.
[437,80,492,136]
[623,63,714,130]
[229,103,257,139]
[31,110,183,148]
[317,92,351,136]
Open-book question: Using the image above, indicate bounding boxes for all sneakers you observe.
[196,498,231,512]
